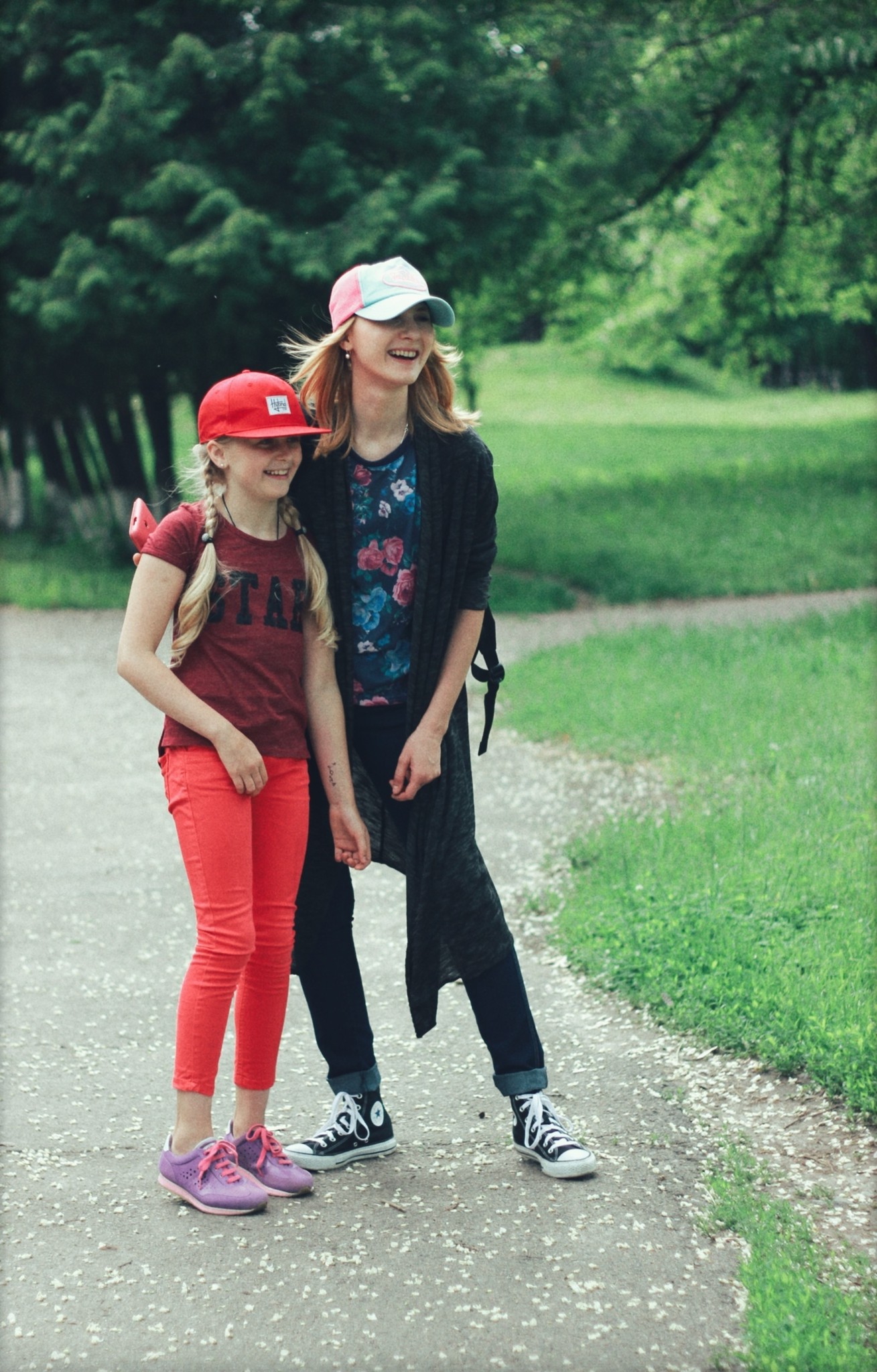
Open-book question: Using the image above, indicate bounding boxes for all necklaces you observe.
[401,420,409,443]
[220,491,280,544]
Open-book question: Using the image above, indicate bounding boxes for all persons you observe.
[117,370,372,1216]
[130,254,600,1180]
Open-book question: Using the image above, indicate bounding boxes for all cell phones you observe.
[129,498,158,552]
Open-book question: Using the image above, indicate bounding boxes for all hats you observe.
[329,256,455,328]
[198,370,333,444]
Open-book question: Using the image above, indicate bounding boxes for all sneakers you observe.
[509,1088,597,1177]
[284,1092,397,1170]
[223,1119,315,1197]
[157,1133,268,1215]
